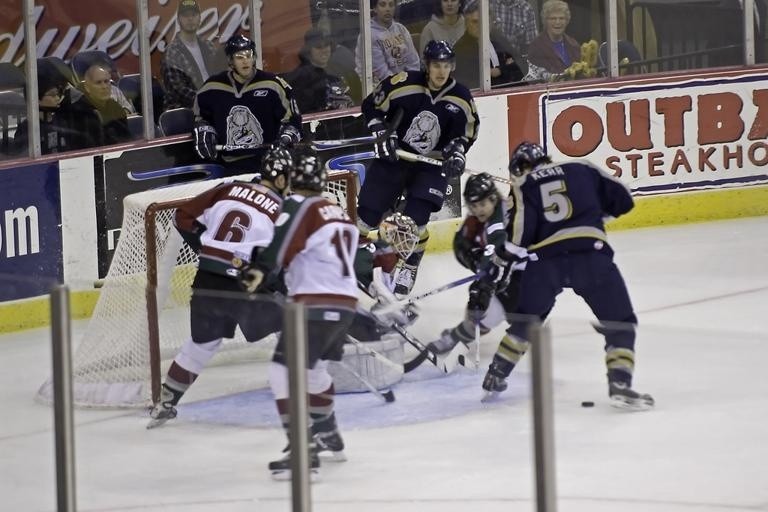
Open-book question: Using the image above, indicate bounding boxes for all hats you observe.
[178,0,198,14]
[303,25,332,49]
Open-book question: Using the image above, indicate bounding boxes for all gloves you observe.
[441,135,471,177]
[190,119,221,160]
[484,247,513,293]
[367,116,398,163]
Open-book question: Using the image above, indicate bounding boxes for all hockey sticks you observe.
[459,268,481,374]
[239,275,396,404]
[214,107,404,151]
[393,322,471,373]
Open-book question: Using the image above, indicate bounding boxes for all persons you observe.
[428,171,507,355]
[280,1,600,178]
[160,2,223,110]
[237,155,359,472]
[354,41,481,300]
[482,140,653,406]
[16,63,137,157]
[149,145,293,420]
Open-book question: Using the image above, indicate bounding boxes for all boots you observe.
[268,429,321,472]
[427,327,471,356]
[609,380,658,406]
[479,360,510,393]
[310,422,344,454]
[393,261,420,296]
[149,382,178,420]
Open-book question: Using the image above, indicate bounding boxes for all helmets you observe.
[508,140,549,178]
[224,35,257,63]
[290,150,326,193]
[380,207,420,263]
[462,171,499,216]
[422,38,457,73]
[259,145,295,187]
